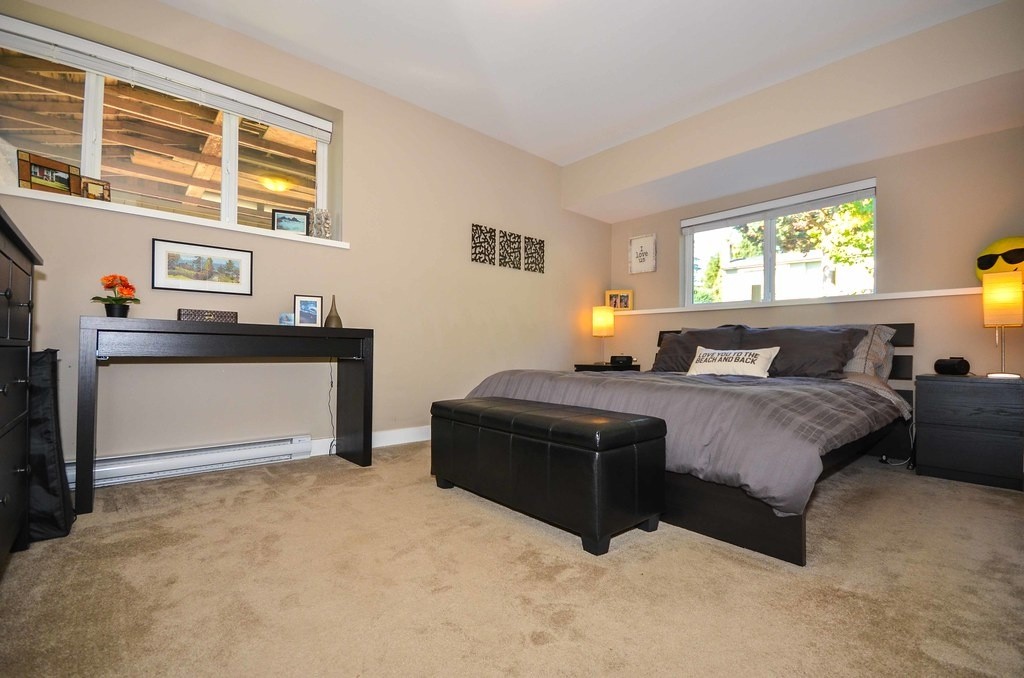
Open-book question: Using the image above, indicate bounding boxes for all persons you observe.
[613,296,616,307]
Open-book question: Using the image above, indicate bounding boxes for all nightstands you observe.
[913,373,1024,491]
[574,362,641,372]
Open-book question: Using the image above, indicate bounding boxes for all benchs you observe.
[429,395,669,557]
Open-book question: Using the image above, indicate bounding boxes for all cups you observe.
[752,285,761,302]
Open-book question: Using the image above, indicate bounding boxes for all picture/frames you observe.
[16,150,81,197]
[604,289,634,311]
[151,238,253,296]
[271,209,310,235]
[80,175,111,202]
[628,232,657,275]
[294,295,323,327]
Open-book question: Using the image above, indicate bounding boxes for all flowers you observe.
[90,274,141,305]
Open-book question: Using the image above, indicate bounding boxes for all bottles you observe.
[324,294,342,328]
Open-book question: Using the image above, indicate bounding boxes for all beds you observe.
[465,321,916,568]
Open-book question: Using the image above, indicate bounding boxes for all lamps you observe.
[592,306,616,364]
[983,271,1024,379]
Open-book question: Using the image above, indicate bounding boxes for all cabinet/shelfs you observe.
[0,206,44,573]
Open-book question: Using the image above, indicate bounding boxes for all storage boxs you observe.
[177,309,238,324]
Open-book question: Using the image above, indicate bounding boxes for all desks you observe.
[72,314,375,515]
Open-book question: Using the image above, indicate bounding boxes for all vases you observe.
[324,295,342,327]
[105,304,130,318]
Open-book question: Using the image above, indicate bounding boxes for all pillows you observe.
[875,341,895,383]
[686,345,780,378]
[679,327,704,333]
[730,324,867,381]
[831,324,897,376]
[651,327,735,371]
